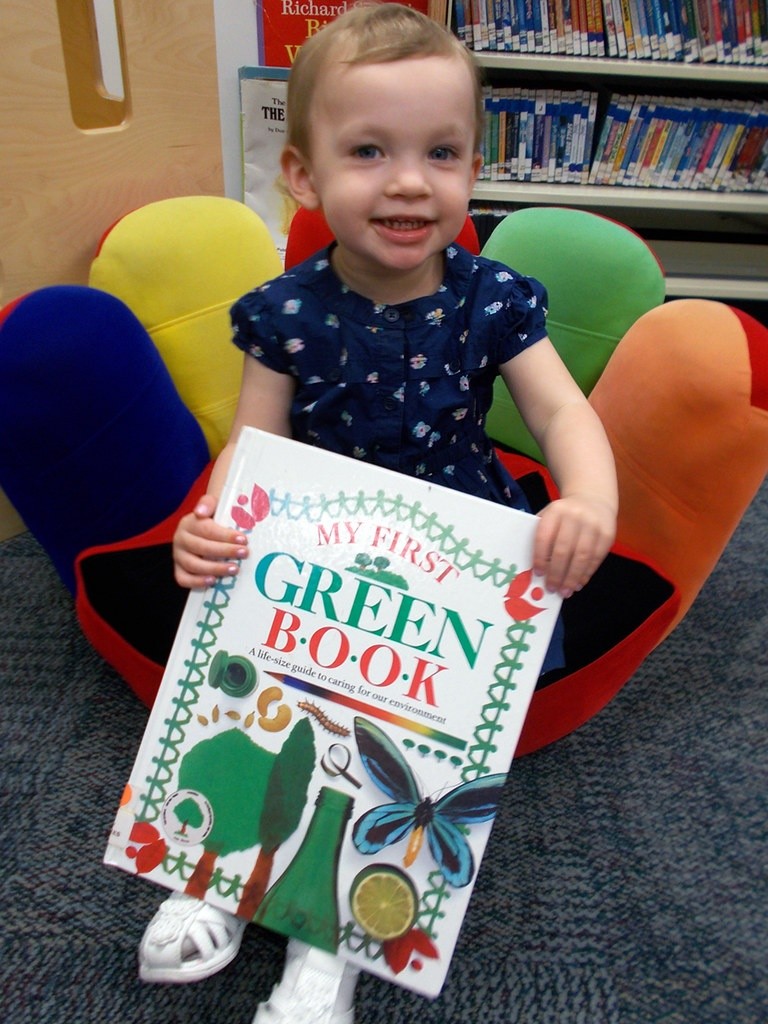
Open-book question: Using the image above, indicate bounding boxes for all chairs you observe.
[0,197,767,761]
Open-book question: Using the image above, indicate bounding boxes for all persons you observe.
[135,1,619,1024]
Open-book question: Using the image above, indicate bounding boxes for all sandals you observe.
[253,938,361,1024]
[138,889,248,984]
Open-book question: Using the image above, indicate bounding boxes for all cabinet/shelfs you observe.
[428,0,768,303]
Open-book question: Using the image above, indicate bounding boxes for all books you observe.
[473,82,768,200]
[99,426,565,1003]
[442,0,768,68]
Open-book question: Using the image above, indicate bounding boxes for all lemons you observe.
[349,863,419,939]
